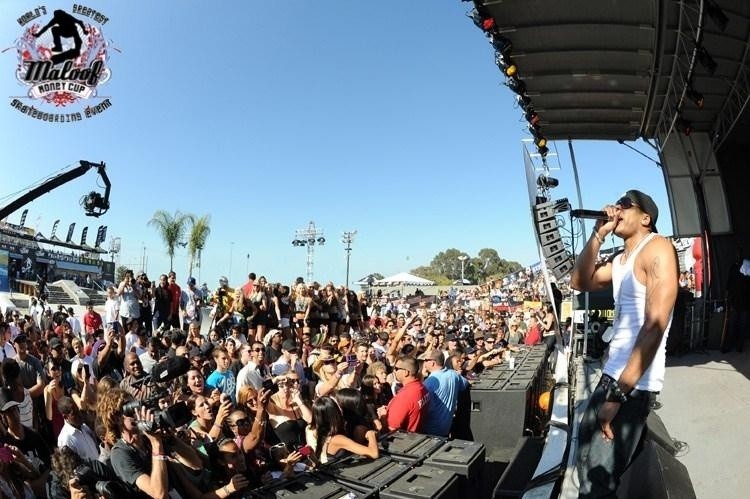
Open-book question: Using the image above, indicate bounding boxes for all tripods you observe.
[207,298,229,337]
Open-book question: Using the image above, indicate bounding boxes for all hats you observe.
[619,190,660,233]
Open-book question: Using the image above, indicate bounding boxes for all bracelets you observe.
[592,226,605,246]
[609,380,627,403]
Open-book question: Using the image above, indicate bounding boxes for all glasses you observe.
[0,268,556,496]
[615,197,641,209]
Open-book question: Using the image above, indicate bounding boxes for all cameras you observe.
[346,354,358,363]
[128,277,136,285]
[73,463,123,498]
[83,364,90,379]
[297,445,314,462]
[262,378,279,396]
[219,288,226,297]
[112,322,118,334]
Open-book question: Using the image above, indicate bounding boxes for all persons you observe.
[678,266,696,295]
[33,9,89,51]
[0,253,613,499]
[722,246,750,353]
[570,190,679,499]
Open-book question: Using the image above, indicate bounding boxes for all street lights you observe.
[341,230,357,288]
[292,221,325,284]
[457,255,467,279]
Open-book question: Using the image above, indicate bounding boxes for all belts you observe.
[601,374,613,391]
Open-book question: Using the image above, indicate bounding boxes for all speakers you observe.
[635,408,678,457]
[532,201,574,281]
[614,439,698,498]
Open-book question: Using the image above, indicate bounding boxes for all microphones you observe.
[570,209,614,221]
